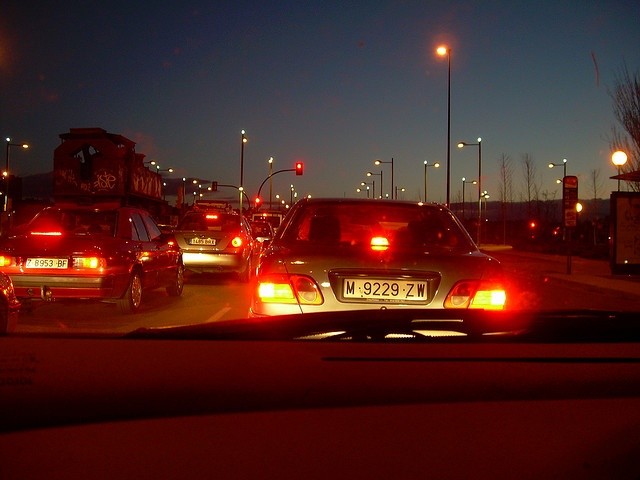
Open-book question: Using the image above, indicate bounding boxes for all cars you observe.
[249,198,513,317]
[174,209,254,282]
[0,207,184,314]
[251,223,273,257]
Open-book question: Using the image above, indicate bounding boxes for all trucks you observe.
[53,128,165,208]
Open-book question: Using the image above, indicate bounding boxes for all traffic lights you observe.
[296,161,305,174]
[210,181,241,192]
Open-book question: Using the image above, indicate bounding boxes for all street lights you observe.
[394,187,405,201]
[423,160,439,201]
[176,177,210,202]
[457,138,485,245]
[366,171,383,200]
[374,157,394,199]
[159,168,174,176]
[610,150,626,190]
[549,158,568,185]
[356,184,370,198]
[436,42,452,209]
[278,185,297,209]
[143,161,156,167]
[240,130,249,185]
[1,135,29,195]
[460,178,477,217]
